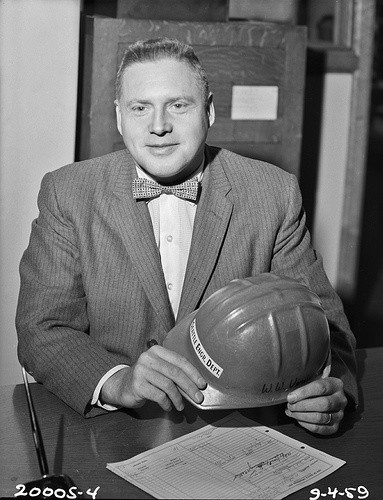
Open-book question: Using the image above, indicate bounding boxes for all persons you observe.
[14,38,359,436]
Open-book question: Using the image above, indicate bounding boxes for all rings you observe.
[324,413,333,425]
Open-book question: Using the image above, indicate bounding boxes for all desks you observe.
[0,347,383,500]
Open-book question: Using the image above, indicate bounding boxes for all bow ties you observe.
[129,177,200,203]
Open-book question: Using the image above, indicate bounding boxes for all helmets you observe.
[161,271,331,412]
